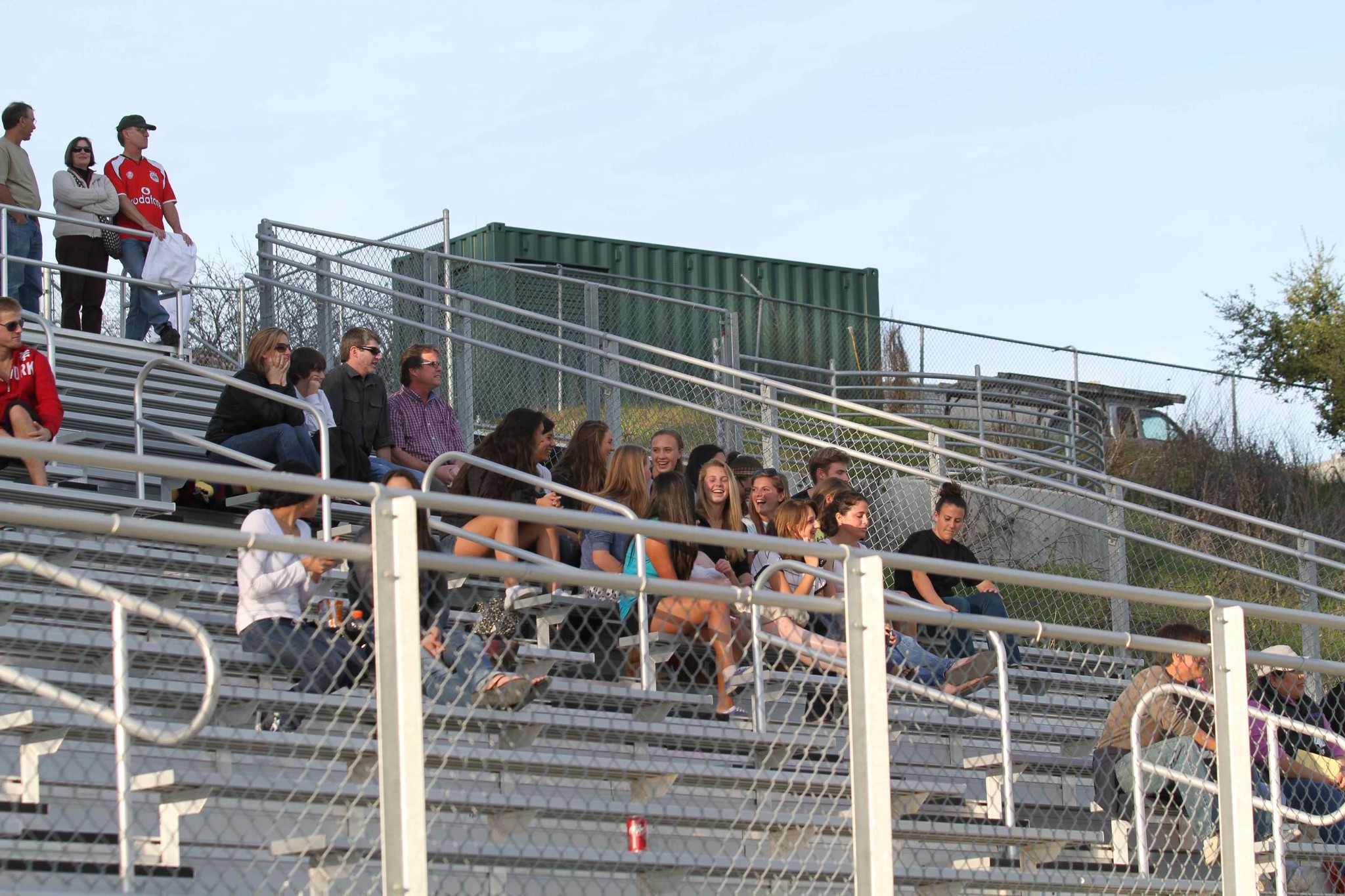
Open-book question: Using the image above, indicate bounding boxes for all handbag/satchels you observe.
[472,597,528,637]
[100,219,122,260]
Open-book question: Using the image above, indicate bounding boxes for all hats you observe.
[116,115,157,131]
[1255,644,1312,676]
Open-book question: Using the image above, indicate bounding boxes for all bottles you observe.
[344,611,366,651]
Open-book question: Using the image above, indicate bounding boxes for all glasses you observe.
[358,346,380,356]
[73,146,92,153]
[129,128,150,134]
[421,362,441,368]
[274,344,292,353]
[0,320,24,331]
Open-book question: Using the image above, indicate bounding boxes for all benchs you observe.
[0,320,1345,896]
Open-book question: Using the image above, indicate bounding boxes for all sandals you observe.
[898,669,915,680]
[946,650,997,686]
[940,676,992,697]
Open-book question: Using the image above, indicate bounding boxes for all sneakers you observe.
[725,662,767,696]
[716,706,752,721]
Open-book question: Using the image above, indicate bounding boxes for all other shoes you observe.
[160,326,179,345]
[471,675,531,706]
[1203,834,1221,865]
[504,584,543,610]
[551,590,585,599]
[1255,828,1302,855]
[501,674,553,712]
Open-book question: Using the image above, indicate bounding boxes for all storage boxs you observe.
[392,221,880,428]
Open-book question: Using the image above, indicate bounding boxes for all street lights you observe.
[1215,369,1239,458]
[1053,345,1081,437]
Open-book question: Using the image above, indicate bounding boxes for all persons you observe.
[579,429,1033,722]
[0,294,64,488]
[548,420,616,533]
[204,327,349,503]
[1094,621,1345,896]
[234,462,369,736]
[320,327,425,494]
[438,407,580,612]
[52,136,119,334]
[0,102,42,324]
[895,481,1032,671]
[385,343,467,496]
[102,114,193,346]
[349,469,553,712]
[617,472,752,722]
[751,491,847,724]
